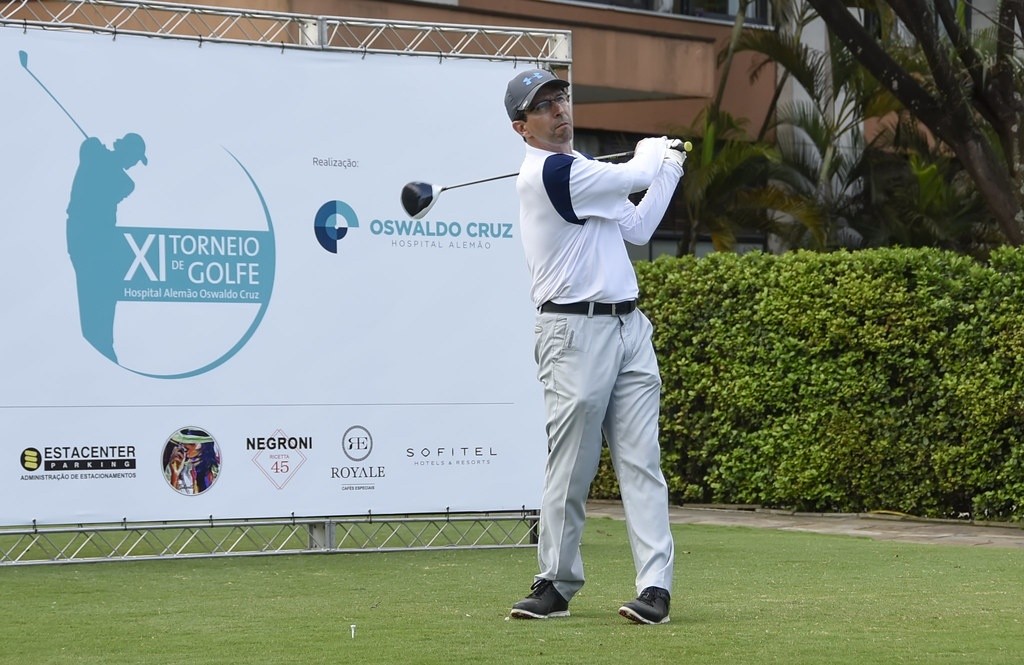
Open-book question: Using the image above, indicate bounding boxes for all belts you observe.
[537,300,638,315]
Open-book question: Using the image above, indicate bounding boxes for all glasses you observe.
[528,93,567,114]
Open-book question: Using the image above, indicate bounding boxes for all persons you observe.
[503,69,686,624]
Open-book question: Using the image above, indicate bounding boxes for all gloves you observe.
[663,138,687,166]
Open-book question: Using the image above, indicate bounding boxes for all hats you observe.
[504,69,570,122]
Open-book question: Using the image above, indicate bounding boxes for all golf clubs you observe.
[399,138,693,221]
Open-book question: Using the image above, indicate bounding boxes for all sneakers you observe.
[618,586,671,624]
[509,578,570,618]
